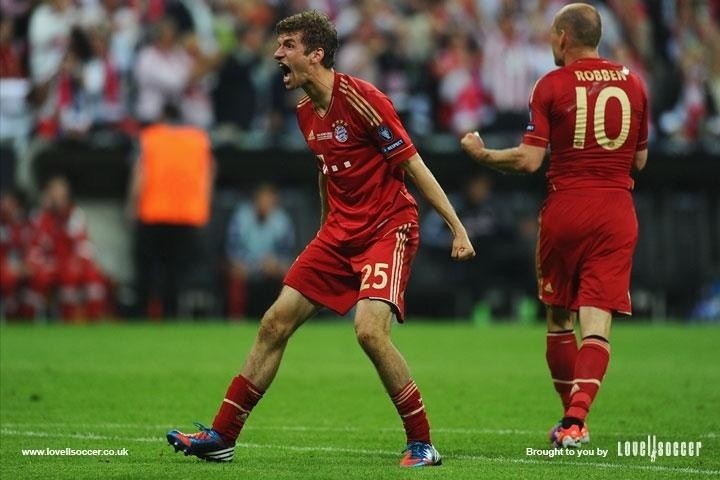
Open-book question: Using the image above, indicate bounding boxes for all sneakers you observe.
[549,421,589,448]
[398,442,442,466]
[168,429,234,462]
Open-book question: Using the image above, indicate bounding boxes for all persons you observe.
[461,3,651,448]
[168,10,477,468]
